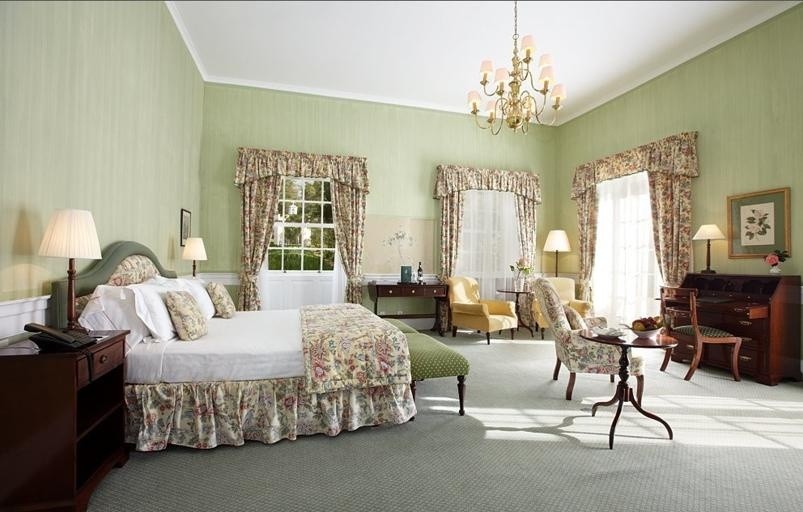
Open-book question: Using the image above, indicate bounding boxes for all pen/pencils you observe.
[93,337,102,338]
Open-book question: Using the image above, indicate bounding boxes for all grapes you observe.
[642,317,656,330]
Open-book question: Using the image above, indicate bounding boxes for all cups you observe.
[400,264,413,285]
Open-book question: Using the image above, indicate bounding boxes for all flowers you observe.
[764,250,790,266]
[508,257,530,279]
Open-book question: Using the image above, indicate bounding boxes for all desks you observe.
[531,278,577,339]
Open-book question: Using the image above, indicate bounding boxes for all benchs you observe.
[382,316,469,421]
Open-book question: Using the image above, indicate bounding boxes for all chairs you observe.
[537,278,644,406]
[659,286,743,382]
[448,277,519,345]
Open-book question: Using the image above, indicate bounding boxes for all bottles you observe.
[417,261,425,284]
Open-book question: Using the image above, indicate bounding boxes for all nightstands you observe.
[0,330,132,512]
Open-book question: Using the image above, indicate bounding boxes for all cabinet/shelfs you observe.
[374,285,448,337]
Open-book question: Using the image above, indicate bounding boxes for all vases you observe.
[512,277,524,290]
[768,266,782,274]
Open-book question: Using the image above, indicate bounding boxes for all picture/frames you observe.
[723,187,792,259]
[181,209,192,245]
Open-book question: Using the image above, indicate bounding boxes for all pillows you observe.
[78,275,238,357]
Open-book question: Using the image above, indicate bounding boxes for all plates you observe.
[595,327,626,339]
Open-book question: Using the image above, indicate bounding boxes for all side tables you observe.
[498,288,537,340]
[580,328,679,449]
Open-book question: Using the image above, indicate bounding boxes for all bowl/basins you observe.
[621,324,667,338]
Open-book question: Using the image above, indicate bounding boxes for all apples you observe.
[634,323,644,330]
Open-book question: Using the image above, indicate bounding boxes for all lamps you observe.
[182,238,209,279]
[472,1,561,135]
[543,229,571,277]
[690,224,726,273]
[38,208,102,335]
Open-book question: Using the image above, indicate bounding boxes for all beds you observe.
[53,242,417,453]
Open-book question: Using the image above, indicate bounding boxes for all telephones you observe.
[24,323,97,351]
